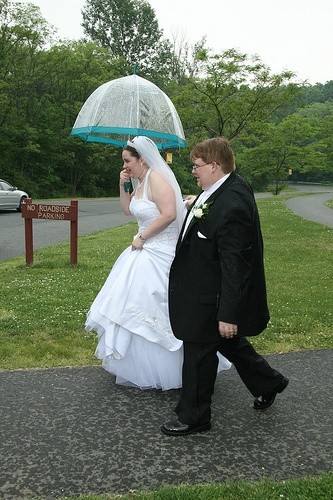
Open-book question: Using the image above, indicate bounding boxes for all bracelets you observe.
[138,233,146,241]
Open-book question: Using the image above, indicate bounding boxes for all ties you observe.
[182,192,207,240]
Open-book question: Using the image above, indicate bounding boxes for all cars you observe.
[0,179,29,213]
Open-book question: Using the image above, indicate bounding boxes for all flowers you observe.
[191,200,213,222]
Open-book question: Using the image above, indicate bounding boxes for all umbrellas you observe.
[69,65,186,192]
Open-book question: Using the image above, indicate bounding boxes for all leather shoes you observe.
[160,416,211,436]
[253,374,289,409]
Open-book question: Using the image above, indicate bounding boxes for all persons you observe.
[161,137,290,436]
[84,136,231,393]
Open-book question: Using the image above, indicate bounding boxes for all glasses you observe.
[193,160,220,171]
